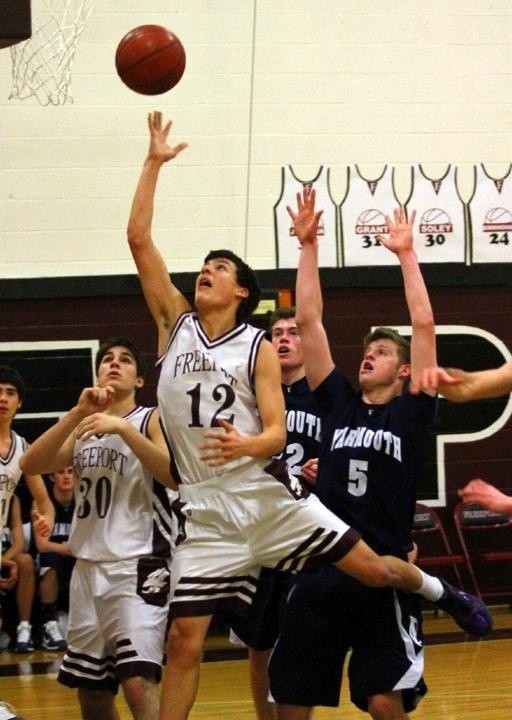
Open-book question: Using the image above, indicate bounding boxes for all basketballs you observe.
[115,25,185,95]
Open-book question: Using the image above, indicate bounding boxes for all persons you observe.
[402,356,511,400]
[280,182,430,720]
[0,363,57,720]
[21,336,177,720]
[124,108,494,720]
[458,472,512,524]
[226,307,321,720]
[0,465,75,652]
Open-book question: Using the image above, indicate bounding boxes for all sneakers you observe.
[41,620,67,650]
[427,577,492,637]
[0,701,25,720]
[15,624,35,652]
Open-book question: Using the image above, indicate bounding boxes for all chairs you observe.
[412,495,511,618]
